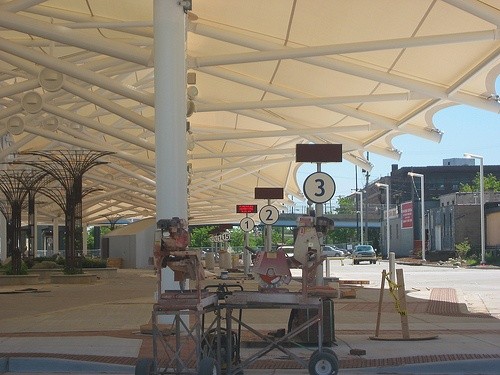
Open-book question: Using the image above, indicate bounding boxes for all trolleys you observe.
[135,284,243,375]
[220,290,340,375]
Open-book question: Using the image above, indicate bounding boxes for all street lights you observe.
[408,172,426,262]
[352,191,364,245]
[375,183,390,260]
[463,153,486,265]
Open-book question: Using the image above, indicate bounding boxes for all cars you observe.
[353,245,378,264]
[324,245,351,257]
[278,246,295,257]
